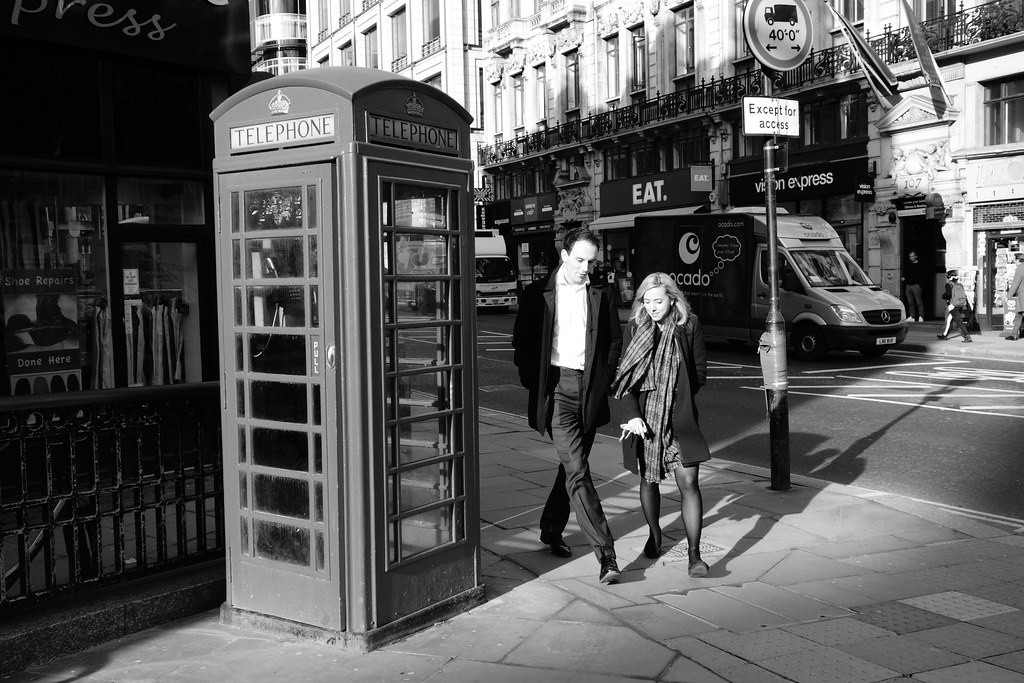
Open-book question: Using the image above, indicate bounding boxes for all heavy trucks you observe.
[392,229,516,314]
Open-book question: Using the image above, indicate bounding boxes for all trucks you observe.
[587,204,908,367]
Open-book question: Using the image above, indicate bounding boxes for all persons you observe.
[511,226,623,582]
[1004,252,1024,340]
[615,271,710,578]
[936,269,972,342]
[900,249,924,322]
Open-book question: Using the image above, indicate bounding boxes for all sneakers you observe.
[906,316,915,322]
[919,316,923,322]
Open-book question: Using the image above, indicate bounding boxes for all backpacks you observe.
[947,282,967,307]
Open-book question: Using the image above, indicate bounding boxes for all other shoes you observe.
[937,334,947,339]
[1005,335,1018,340]
[644,529,662,558]
[962,338,971,342]
[688,561,708,578]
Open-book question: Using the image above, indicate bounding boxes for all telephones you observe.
[262,256,311,312]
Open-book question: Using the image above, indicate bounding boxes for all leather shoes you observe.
[540,530,572,557]
[600,558,620,583]
